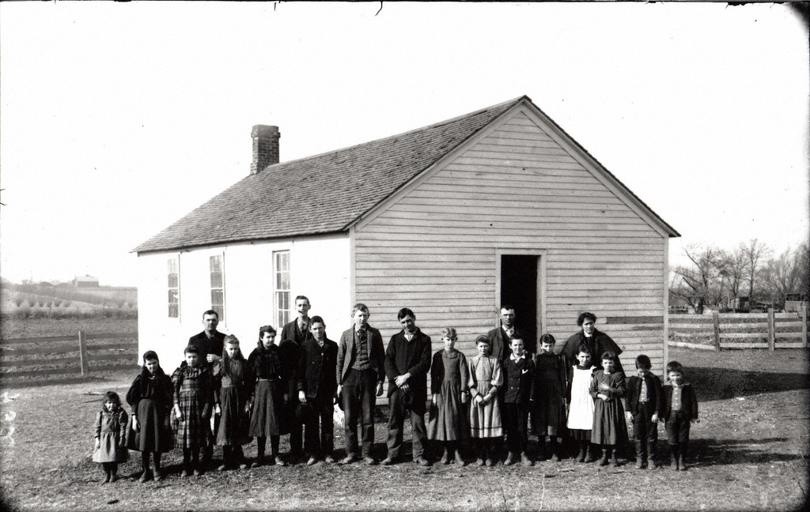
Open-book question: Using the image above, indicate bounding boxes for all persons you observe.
[94,389,129,487]
[125,295,701,479]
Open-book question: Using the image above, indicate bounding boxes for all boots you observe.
[576,439,685,472]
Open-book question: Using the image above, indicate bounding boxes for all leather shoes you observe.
[103,459,248,483]
[414,453,532,467]
[308,456,395,465]
